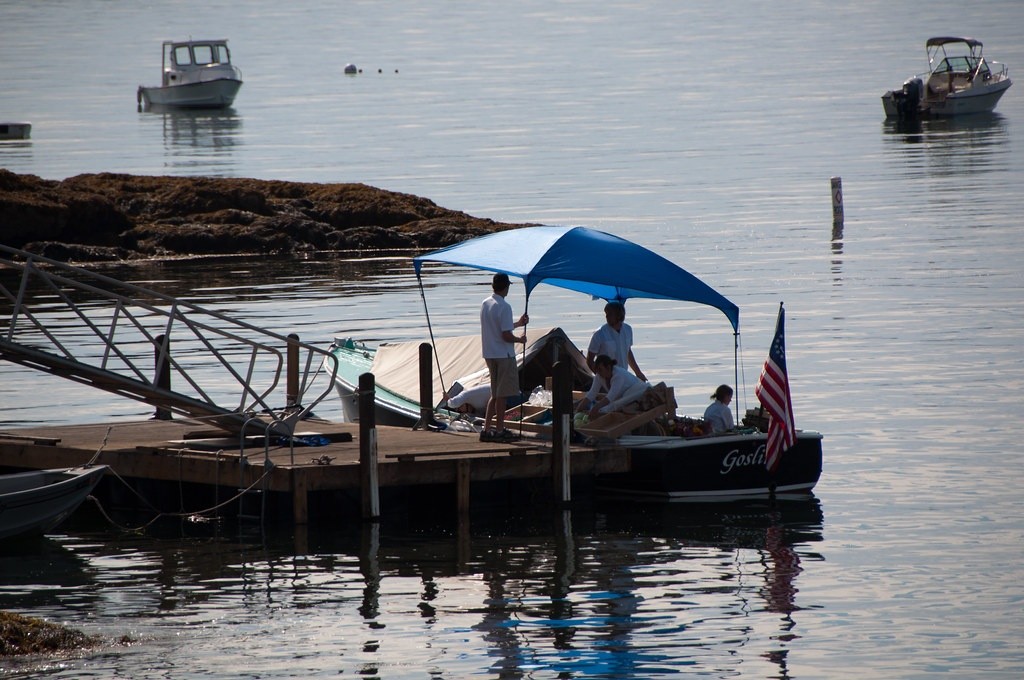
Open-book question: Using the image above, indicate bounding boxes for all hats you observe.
[493,273,512,285]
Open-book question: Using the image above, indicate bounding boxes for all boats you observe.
[324,225,825,507]
[882,36,1013,132]
[137,35,244,106]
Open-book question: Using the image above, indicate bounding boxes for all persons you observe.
[586,302,649,383]
[480,272,529,443]
[576,354,652,420]
[704,385,735,432]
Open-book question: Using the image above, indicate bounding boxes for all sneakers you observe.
[493,430,520,442]
[479,429,497,441]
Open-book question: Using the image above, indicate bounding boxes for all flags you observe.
[754,307,797,474]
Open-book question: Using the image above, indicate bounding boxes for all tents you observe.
[412,225,740,440]
